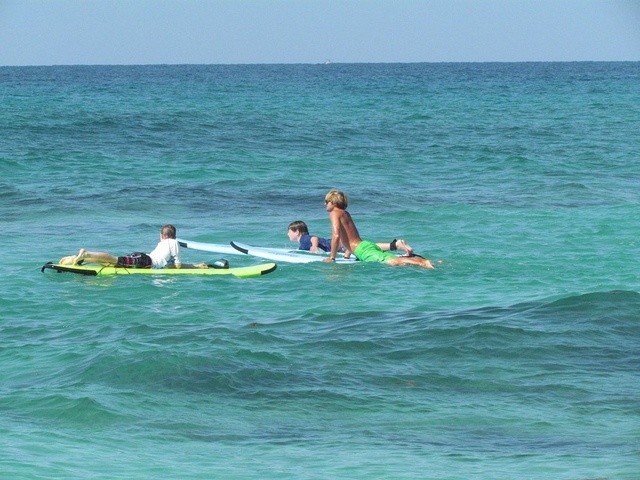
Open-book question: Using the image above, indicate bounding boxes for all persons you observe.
[287,220,413,255]
[321,190,436,269]
[58,224,182,269]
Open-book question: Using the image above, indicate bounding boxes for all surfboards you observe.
[230,240,362,266]
[45,261,278,277]
[176,237,359,259]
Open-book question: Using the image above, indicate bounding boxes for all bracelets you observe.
[330,256,335,261]
